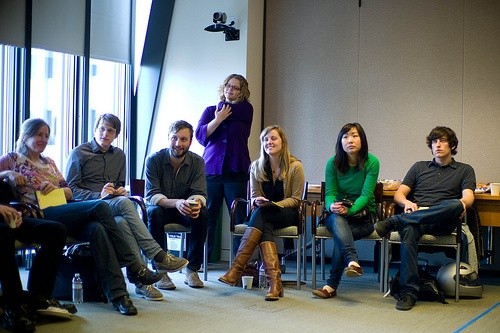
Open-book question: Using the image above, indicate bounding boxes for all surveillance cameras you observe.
[213,12,226,24]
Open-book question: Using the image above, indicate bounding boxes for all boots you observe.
[217,227,263,287]
[259,241,284,301]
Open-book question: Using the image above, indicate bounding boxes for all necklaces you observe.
[270,163,280,174]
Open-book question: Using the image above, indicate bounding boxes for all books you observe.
[100,194,125,200]
[36,188,68,211]
[256,199,285,209]
[417,206,430,210]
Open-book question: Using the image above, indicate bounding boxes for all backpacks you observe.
[383,268,449,302]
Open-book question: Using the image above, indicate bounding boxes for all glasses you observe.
[225,84,241,90]
[96,127,117,133]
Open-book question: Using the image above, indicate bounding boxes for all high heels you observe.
[127,258,167,285]
[112,295,138,315]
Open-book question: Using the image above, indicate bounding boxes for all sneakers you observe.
[184,270,205,287]
[134,284,164,301]
[155,274,176,289]
[154,251,190,272]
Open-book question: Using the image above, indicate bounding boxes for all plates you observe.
[474,189,490,193]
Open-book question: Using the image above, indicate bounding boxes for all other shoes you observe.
[0,307,35,333]
[345,265,363,276]
[396,292,416,310]
[312,287,337,299]
[46,299,77,314]
[373,217,395,238]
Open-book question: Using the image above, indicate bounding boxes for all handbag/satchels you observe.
[318,198,373,226]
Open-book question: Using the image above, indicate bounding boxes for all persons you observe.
[218,125,305,301]
[0,118,163,315]
[194,74,254,272]
[145,120,209,290]
[65,113,189,301]
[312,122,380,299]
[373,126,476,310]
[0,178,77,333]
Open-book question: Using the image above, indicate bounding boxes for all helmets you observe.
[435,261,482,298]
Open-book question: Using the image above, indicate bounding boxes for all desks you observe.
[305,185,500,273]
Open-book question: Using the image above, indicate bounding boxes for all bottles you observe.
[72,274,83,303]
[259,262,267,288]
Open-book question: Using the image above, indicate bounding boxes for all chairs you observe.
[128,178,461,302]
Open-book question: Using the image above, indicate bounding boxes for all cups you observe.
[187,201,199,215]
[242,276,254,290]
[490,183,500,196]
[8,213,17,228]
